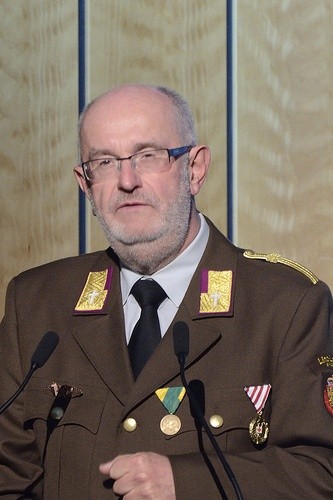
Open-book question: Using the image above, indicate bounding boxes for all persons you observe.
[0,84,332,500]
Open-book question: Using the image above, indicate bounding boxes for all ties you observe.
[126,280,167,383]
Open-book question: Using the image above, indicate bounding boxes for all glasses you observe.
[80,144,196,181]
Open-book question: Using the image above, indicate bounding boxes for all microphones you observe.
[174,322,245,500]
[0,331,60,413]
